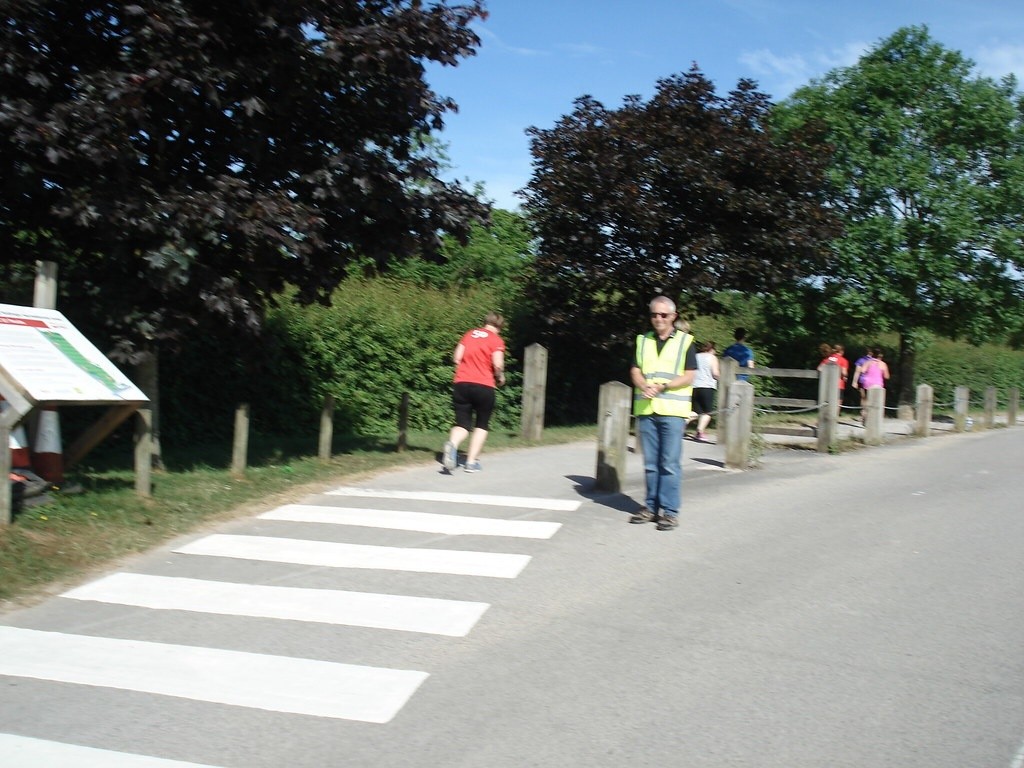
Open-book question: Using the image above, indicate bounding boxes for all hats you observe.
[872,348,882,354]
[735,327,750,335]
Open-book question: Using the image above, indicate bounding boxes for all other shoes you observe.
[696,431,710,440]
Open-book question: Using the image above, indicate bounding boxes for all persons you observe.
[686,341,720,442]
[630,297,700,530]
[721,328,755,381]
[672,319,690,334]
[441,313,506,477]
[816,344,890,428]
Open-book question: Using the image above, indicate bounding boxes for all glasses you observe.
[649,311,671,319]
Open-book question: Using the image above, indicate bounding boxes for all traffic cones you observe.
[33,403,61,489]
[0,395,33,474]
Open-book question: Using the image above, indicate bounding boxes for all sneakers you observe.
[655,514,680,530]
[442,440,458,471]
[629,505,657,524]
[463,461,482,472]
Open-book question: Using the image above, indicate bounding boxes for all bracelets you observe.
[663,383,668,394]
